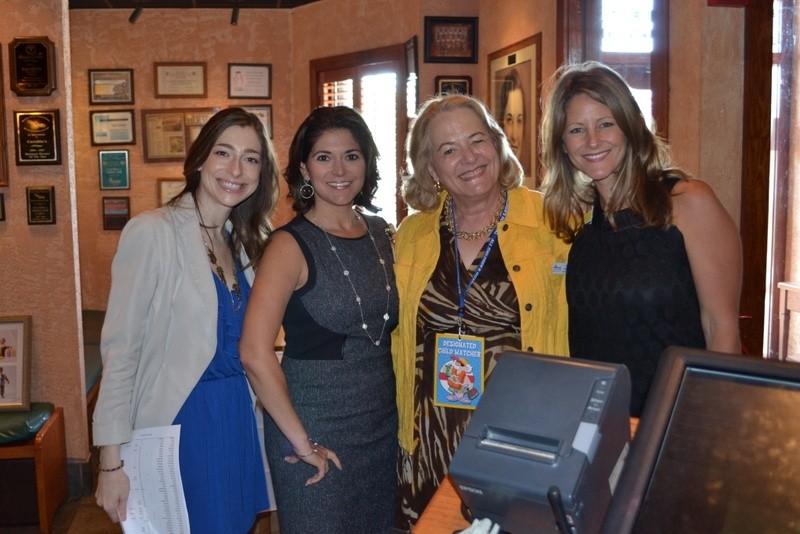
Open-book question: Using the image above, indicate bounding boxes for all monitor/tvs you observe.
[598,345,800,534]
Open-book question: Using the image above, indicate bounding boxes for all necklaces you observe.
[309,210,390,345]
[443,191,506,239]
[203,239,243,311]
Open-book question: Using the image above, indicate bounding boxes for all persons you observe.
[239,105,400,534]
[89,107,280,534]
[391,96,582,529]
[538,61,742,420]
[499,68,526,162]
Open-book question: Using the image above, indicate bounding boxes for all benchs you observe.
[1,402,67,534]
[84,309,108,412]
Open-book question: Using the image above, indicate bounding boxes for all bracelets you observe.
[98,460,124,472]
[295,438,319,457]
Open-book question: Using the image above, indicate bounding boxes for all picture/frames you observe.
[0,315,31,410]
[486,32,542,190]
[435,76,472,96]
[424,14,478,64]
